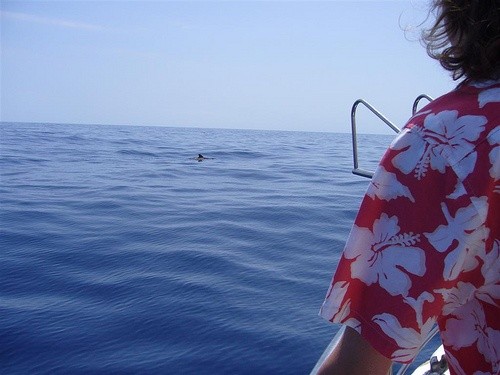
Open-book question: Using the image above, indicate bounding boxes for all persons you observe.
[310,1,499,374]
